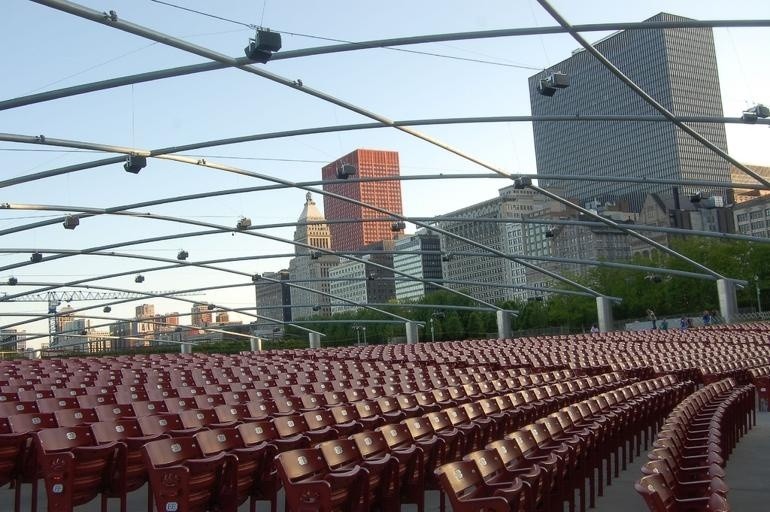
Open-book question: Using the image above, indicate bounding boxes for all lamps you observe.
[252,274,261,281]
[30,253,42,263]
[177,250,188,260]
[687,191,705,203]
[123,156,146,174]
[8,278,17,285]
[63,216,79,229]
[310,251,321,259]
[244,29,281,64]
[536,70,569,97]
[513,176,532,189]
[545,224,555,237]
[103,306,111,312]
[135,276,144,283]
[740,103,770,121]
[334,163,357,179]
[236,217,251,230]
[391,222,405,232]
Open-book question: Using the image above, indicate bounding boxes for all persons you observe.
[679,308,721,329]
[659,319,669,330]
[590,323,599,333]
[647,308,657,330]
